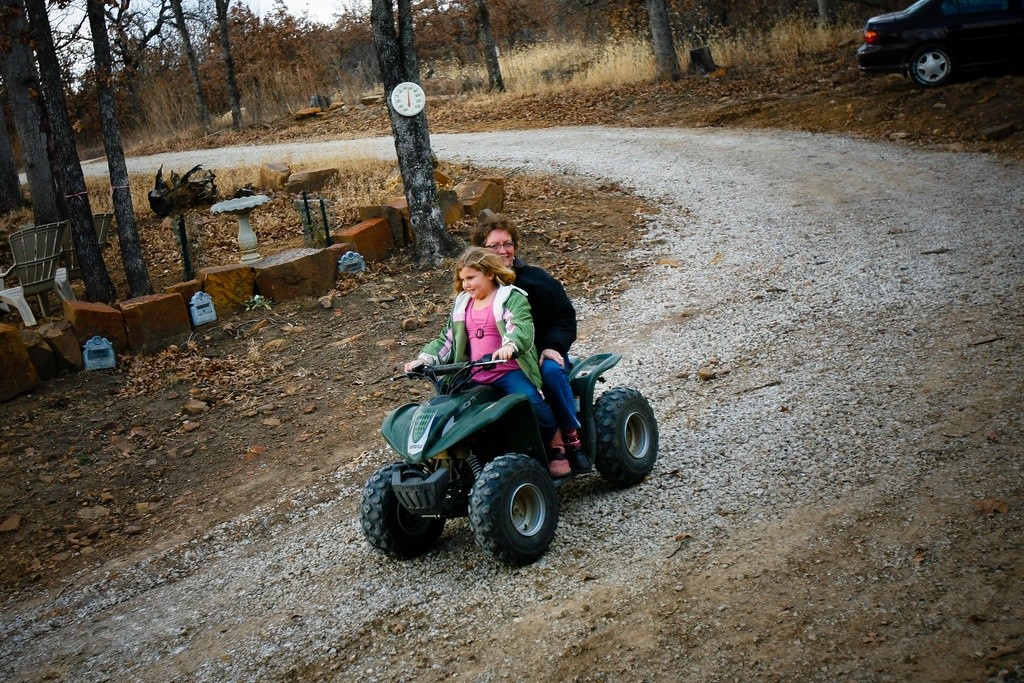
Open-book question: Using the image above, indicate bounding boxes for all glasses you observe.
[484,241,515,249]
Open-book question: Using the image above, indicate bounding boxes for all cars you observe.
[857,0,1024,90]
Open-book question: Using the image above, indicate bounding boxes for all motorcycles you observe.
[358,351,660,574]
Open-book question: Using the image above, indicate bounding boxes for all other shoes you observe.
[549,459,572,477]
[569,447,591,473]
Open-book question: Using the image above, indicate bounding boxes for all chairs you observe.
[0,213,114,327]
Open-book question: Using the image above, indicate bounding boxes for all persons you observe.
[436,216,591,473]
[405,247,571,478]
